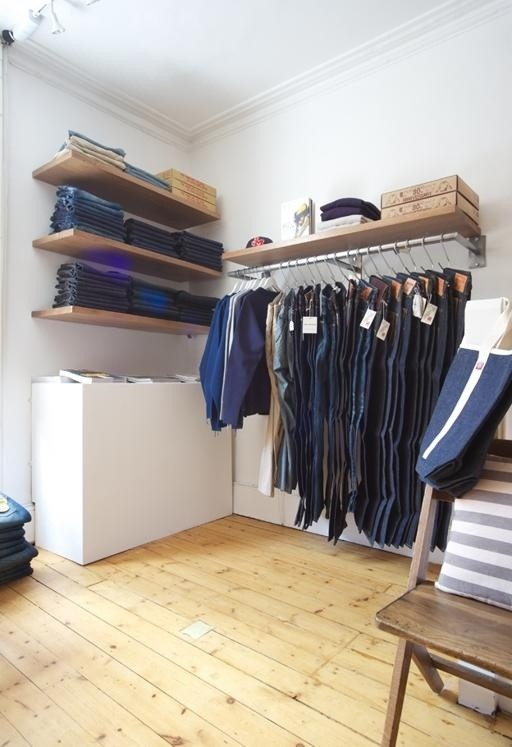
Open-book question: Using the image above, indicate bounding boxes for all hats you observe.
[246,237,273,248]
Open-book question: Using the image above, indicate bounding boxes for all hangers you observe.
[217,233,487,310]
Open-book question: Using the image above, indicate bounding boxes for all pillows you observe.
[435,454,512,611]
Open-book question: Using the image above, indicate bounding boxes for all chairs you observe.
[374,452,511,746]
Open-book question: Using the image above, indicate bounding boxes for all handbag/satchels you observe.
[415,298,512,499]
